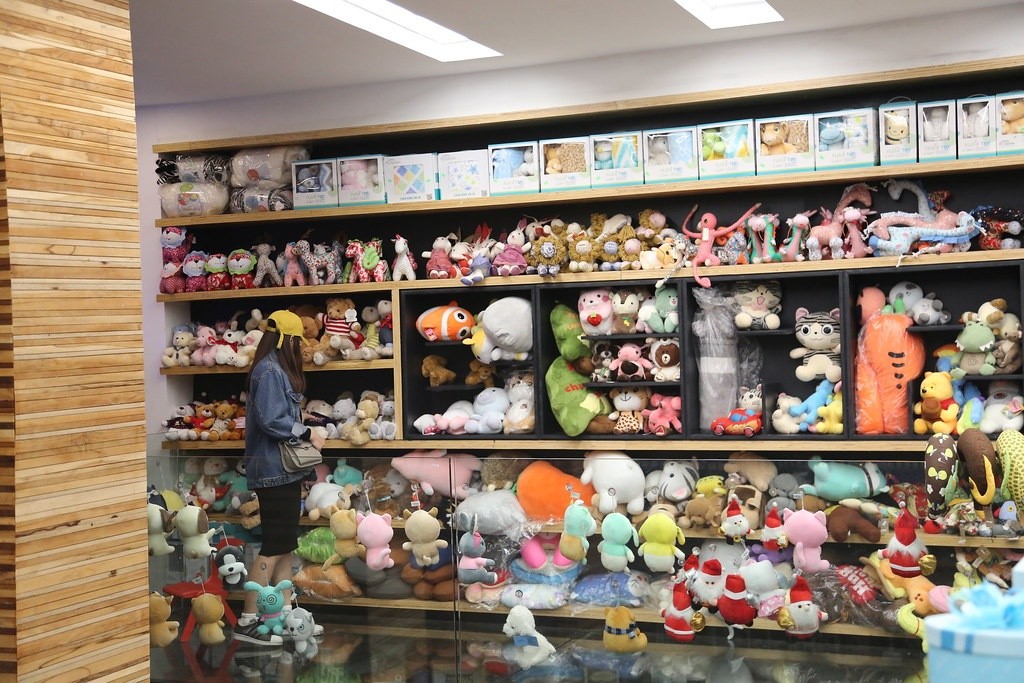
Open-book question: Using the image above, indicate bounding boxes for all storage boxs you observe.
[386,151,440,206]
[589,130,645,190]
[438,148,489,199]
[541,136,591,193]
[644,121,700,185]
[291,158,339,209]
[996,92,1024,158]
[878,101,918,168]
[814,107,882,172]
[918,101,959,162]
[489,140,541,197]
[756,113,817,178]
[957,97,996,161]
[700,117,757,182]
[338,153,386,207]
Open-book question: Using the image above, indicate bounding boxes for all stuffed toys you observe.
[293,632,803,683]
[146,94,1024,670]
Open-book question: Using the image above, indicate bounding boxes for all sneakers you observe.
[233,619,283,645]
[283,624,325,635]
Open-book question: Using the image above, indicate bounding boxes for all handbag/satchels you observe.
[278,440,323,473]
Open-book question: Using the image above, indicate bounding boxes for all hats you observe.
[265,310,311,349]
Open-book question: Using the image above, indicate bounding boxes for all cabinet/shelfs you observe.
[152,53,1024,640]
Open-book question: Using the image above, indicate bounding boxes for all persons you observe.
[234,639,296,683]
[234,309,325,645]
